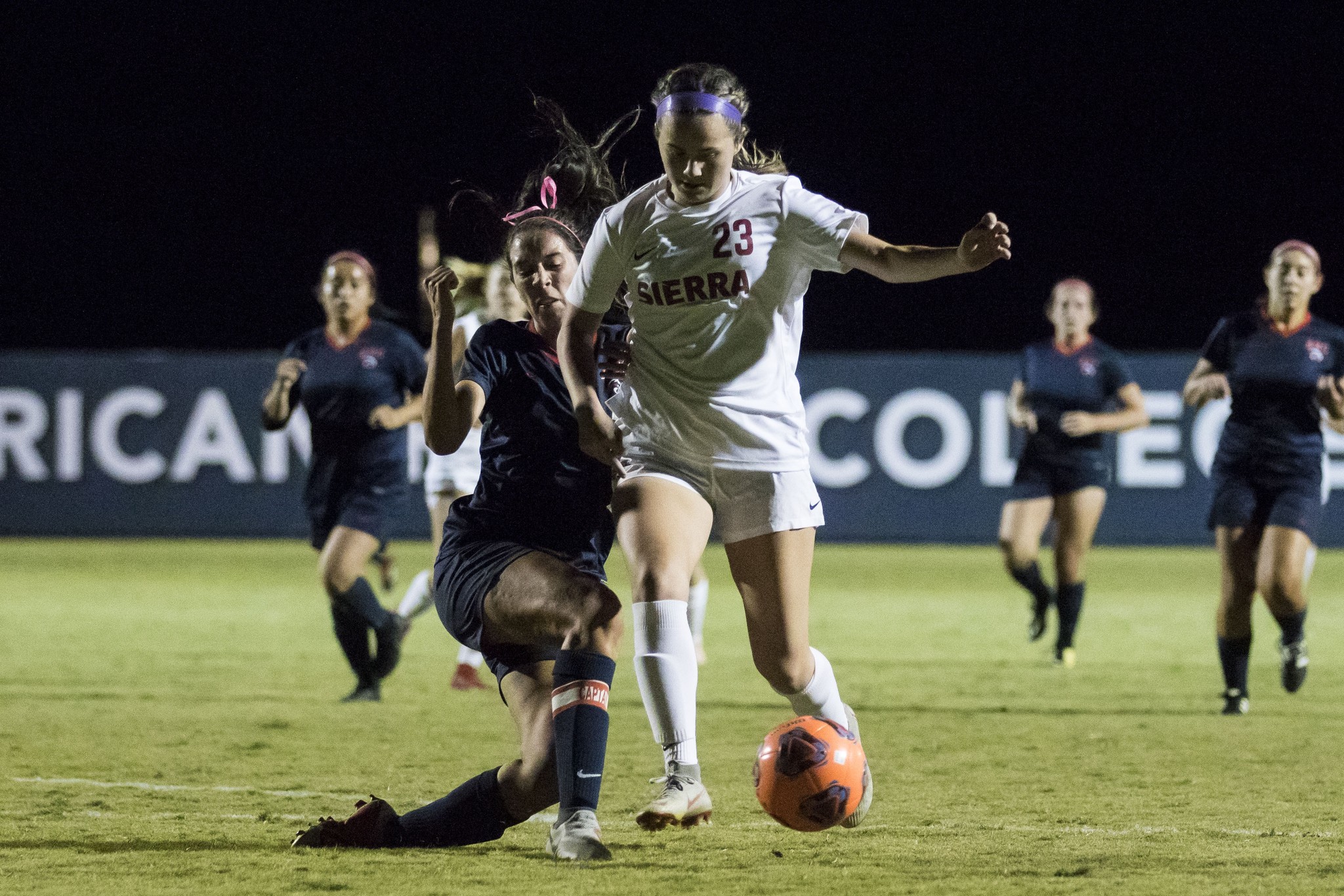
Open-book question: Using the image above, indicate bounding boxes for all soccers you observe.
[753,714,868,832]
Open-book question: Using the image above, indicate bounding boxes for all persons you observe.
[999,274,1153,657]
[1185,240,1344,717]
[555,62,1014,829]
[259,250,435,703]
[291,174,633,861]
[384,247,537,690]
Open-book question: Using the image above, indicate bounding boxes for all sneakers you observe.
[1281,633,1308,694]
[1028,588,1052,640]
[290,794,403,848]
[837,702,873,828]
[452,664,486,690]
[374,612,409,678]
[1222,687,1249,715]
[344,676,380,703]
[546,809,611,861]
[636,773,712,832]
[1054,631,1073,660]
